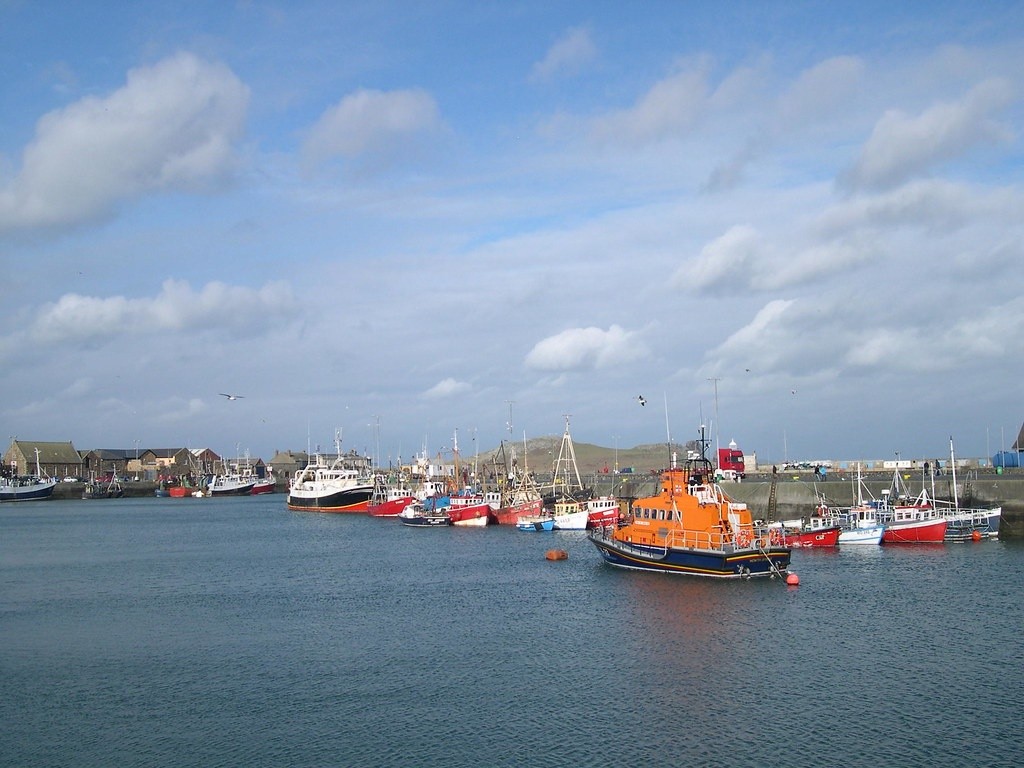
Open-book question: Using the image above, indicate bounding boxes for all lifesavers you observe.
[770,529,780,545]
[815,535,820,541]
[818,505,829,517]
[803,540,813,548]
[736,529,751,548]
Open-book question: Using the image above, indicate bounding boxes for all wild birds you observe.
[219,394,244,401]
[792,389,797,394]
[638,396,647,406]
[745,369,751,373]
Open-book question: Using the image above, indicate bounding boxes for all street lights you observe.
[612,435,620,470]
[9,436,17,476]
[133,440,141,476]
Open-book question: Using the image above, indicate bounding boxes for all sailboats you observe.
[445,401,544,527]
[936,435,1002,540]
[552,414,621,530]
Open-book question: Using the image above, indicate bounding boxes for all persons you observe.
[935,459,940,476]
[740,472,746,480]
[18,477,25,486]
[772,465,776,475]
[815,464,827,482]
[924,461,929,476]
[784,462,790,470]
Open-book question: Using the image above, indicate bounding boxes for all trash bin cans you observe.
[996,466,1003,474]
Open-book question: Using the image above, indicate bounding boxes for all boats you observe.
[412,434,464,512]
[587,390,792,576]
[399,500,450,527]
[752,459,947,548]
[82,463,123,499]
[552,504,588,530]
[517,516,555,532]
[367,442,419,517]
[155,442,277,498]
[0,447,57,503]
[286,427,387,513]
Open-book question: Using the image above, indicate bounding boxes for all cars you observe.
[63,477,78,483]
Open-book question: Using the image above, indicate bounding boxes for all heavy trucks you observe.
[665,449,745,479]
